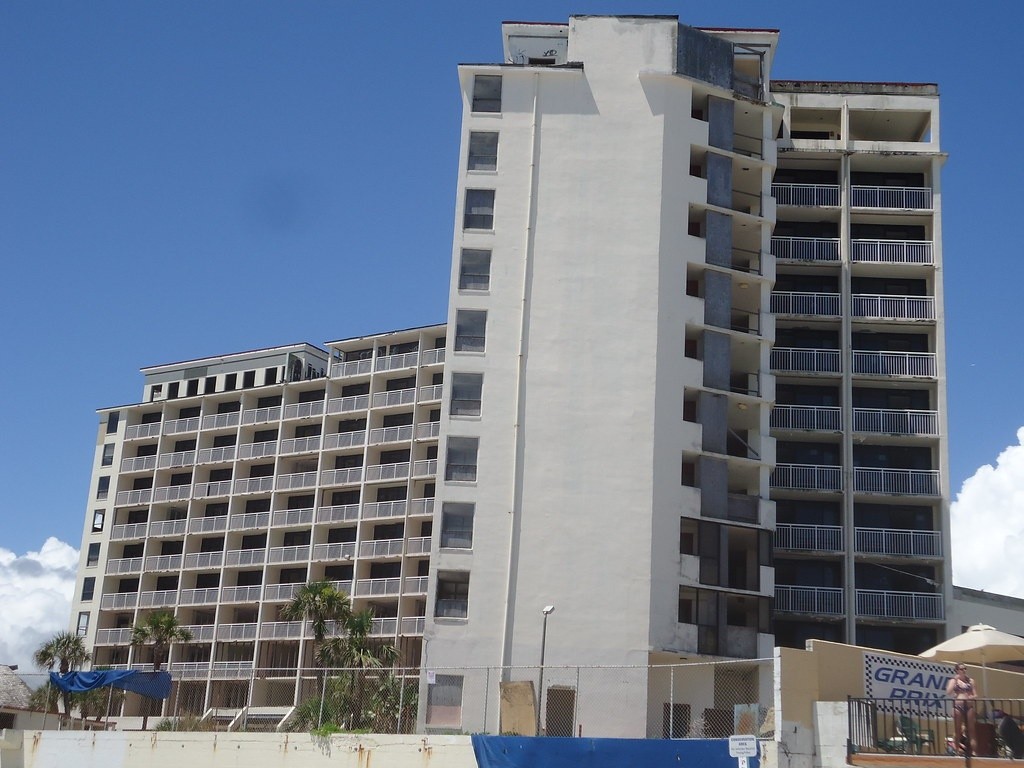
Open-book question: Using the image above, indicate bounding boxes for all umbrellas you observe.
[918,622,1024,697]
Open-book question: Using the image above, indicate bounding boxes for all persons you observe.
[979,697,1006,719]
[946,662,978,756]
[896,716,923,754]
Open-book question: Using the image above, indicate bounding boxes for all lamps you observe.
[740,282,748,289]
[737,403,747,411]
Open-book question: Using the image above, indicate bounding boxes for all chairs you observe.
[896,715,936,755]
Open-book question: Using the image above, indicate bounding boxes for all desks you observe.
[889,734,930,754]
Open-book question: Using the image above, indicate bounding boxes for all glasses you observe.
[957,668,966,671]
